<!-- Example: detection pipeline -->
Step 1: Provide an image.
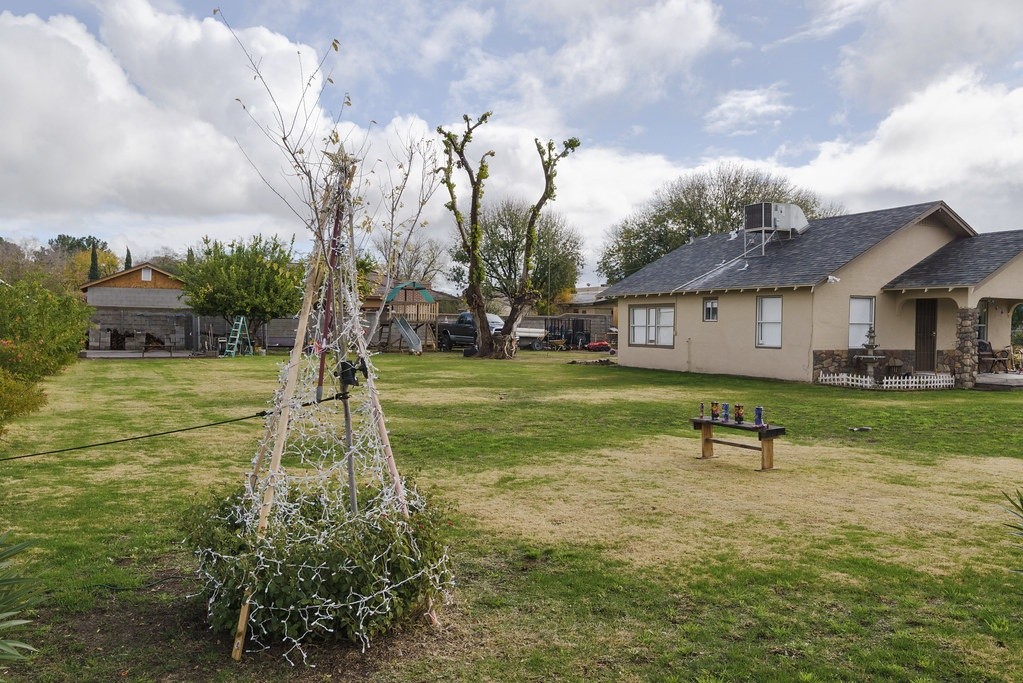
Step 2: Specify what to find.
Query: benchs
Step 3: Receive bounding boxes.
[139,345,176,358]
[689,416,785,470]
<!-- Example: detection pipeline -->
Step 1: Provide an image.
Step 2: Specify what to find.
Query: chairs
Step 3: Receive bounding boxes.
[978,339,1008,374]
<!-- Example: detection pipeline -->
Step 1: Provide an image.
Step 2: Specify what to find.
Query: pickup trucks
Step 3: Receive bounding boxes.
[430,312,519,357]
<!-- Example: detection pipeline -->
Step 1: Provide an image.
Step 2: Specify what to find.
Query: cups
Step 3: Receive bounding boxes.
[721,403,730,422]
[711,402,720,421]
[735,404,744,424]
[754,406,763,425]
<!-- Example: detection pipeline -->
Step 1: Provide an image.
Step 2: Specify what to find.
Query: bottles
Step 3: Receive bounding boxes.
[699,402,704,420]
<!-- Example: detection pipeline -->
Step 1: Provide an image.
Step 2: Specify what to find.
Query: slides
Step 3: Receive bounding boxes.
[392,316,422,350]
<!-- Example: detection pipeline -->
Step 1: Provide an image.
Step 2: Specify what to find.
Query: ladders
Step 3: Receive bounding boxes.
[223,315,252,358]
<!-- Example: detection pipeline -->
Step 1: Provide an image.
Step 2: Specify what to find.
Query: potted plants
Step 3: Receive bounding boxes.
[944,352,955,378]
[885,357,903,376]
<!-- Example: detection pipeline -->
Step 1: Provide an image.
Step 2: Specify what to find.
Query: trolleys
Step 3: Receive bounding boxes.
[549,338,570,351]
